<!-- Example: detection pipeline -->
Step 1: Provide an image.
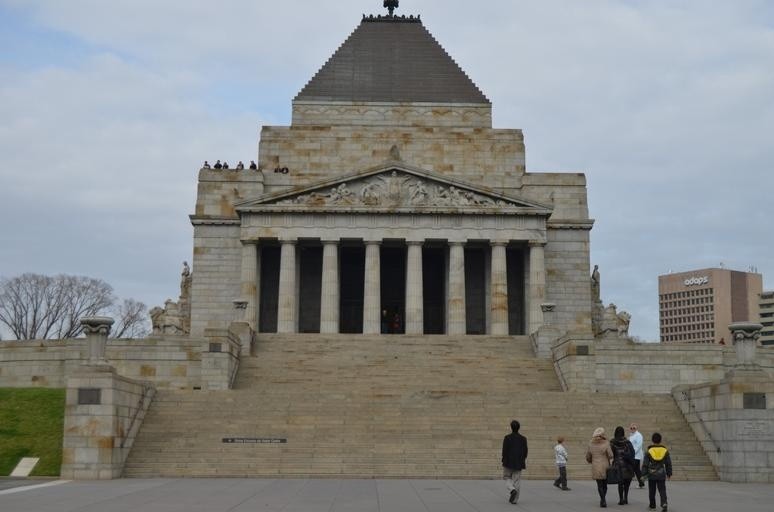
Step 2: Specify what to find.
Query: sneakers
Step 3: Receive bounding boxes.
[509,489,518,504]
[552,482,572,491]
[617,498,629,506]
[661,504,668,512]
[648,504,656,511]
[599,499,608,508]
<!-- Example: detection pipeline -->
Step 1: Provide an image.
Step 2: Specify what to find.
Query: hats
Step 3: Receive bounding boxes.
[593,427,605,438]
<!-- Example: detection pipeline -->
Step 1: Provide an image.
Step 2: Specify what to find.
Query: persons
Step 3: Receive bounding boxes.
[380,308,389,334]
[641,432,673,509]
[609,425,636,505]
[501,419,528,504]
[293,171,517,209]
[390,311,401,330]
[626,422,645,489]
[585,426,615,508]
[202,160,289,174]
[180,261,189,295]
[591,265,601,303]
[553,436,572,492]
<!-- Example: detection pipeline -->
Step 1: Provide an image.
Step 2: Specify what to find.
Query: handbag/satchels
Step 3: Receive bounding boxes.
[605,456,622,485]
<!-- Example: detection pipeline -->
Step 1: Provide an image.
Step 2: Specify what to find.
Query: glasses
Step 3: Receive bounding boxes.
[630,426,636,430]
